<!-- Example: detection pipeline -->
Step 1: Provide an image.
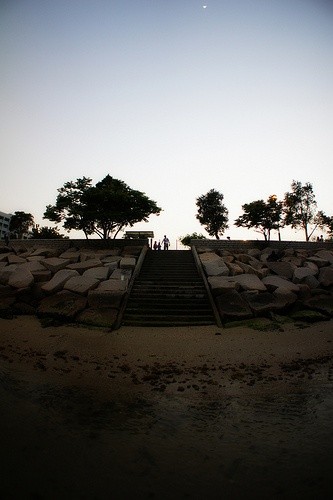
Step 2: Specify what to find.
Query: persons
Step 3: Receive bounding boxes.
[157,242,161,250]
[153,241,157,250]
[161,235,170,252]
